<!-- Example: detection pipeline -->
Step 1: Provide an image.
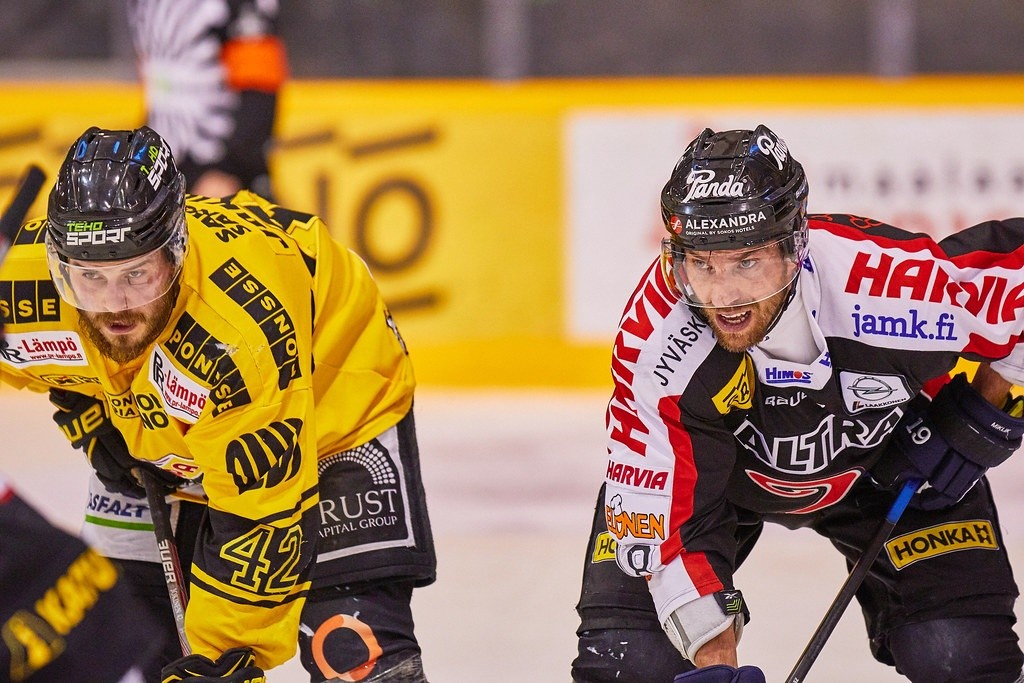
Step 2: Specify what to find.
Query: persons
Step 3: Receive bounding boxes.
[0,126,438,683]
[571,124,1024,682]
[126,0,289,205]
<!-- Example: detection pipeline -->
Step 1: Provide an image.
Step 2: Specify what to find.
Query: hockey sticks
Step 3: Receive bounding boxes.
[784,479,917,683]
[142,464,189,657]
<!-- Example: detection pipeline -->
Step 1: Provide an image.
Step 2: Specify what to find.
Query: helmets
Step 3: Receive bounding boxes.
[47,125,187,260]
[661,124,809,250]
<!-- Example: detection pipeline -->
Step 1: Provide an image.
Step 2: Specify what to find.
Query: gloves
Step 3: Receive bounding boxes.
[160,647,266,683]
[674,664,766,683]
[870,372,1024,511]
[48,387,190,499]
